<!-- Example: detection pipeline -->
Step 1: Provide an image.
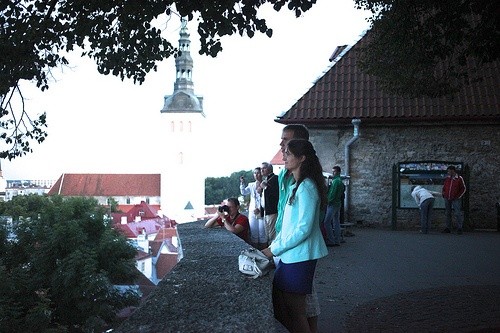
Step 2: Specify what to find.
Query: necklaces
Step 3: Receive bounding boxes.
[230,212,240,224]
[267,174,275,182]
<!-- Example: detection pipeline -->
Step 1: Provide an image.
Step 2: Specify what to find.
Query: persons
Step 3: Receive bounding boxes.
[240,162,279,269]
[411,185,435,234]
[261,125,327,333]
[325,165,345,247]
[442,165,467,235]
[205,198,249,242]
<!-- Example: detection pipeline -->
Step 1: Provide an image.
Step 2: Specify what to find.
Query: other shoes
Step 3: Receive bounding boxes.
[445,227,451,233]
[419,228,428,234]
[457,228,462,234]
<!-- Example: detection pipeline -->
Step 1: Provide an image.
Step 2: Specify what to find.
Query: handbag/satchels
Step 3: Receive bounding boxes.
[237,248,271,279]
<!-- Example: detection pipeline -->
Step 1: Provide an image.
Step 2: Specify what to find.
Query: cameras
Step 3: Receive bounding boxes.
[218,205,229,213]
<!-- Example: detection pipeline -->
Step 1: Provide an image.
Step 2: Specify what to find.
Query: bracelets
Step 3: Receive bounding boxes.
[222,219,227,222]
[264,184,268,190]
